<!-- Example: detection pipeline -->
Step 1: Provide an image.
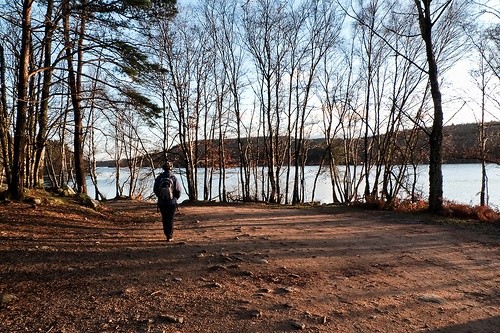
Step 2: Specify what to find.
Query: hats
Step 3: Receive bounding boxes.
[163,162,173,170]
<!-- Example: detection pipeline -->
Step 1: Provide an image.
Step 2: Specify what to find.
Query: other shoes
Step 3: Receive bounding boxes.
[167,236,174,242]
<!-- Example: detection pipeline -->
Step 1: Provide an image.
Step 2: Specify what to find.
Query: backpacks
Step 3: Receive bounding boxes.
[159,173,174,200]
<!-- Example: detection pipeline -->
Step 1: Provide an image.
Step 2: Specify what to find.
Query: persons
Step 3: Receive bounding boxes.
[153,160,181,241]
